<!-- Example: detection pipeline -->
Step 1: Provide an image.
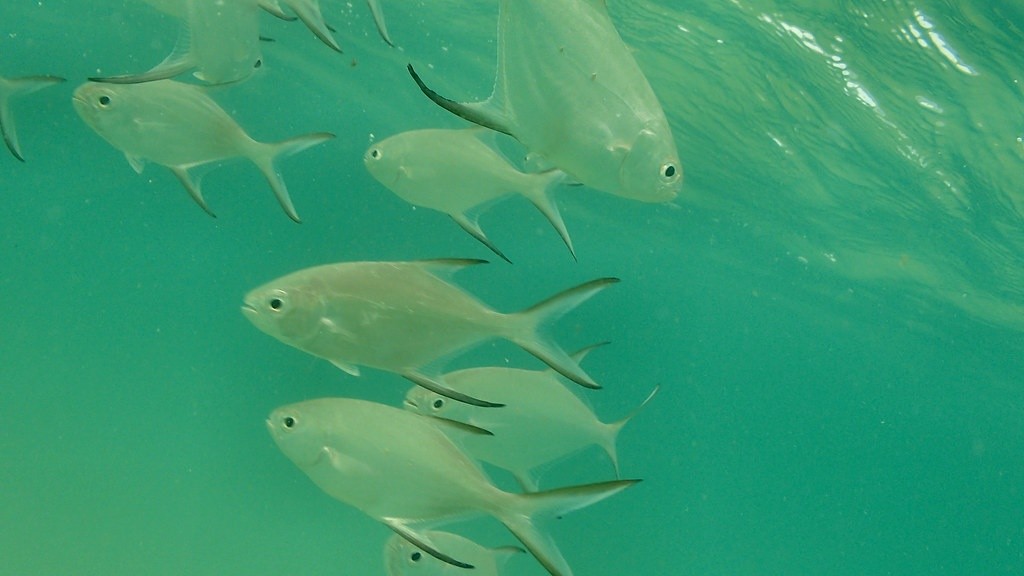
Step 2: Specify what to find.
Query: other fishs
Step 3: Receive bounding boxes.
[242,258,659,576]
[364,0,682,266]
[0,0,396,222]
